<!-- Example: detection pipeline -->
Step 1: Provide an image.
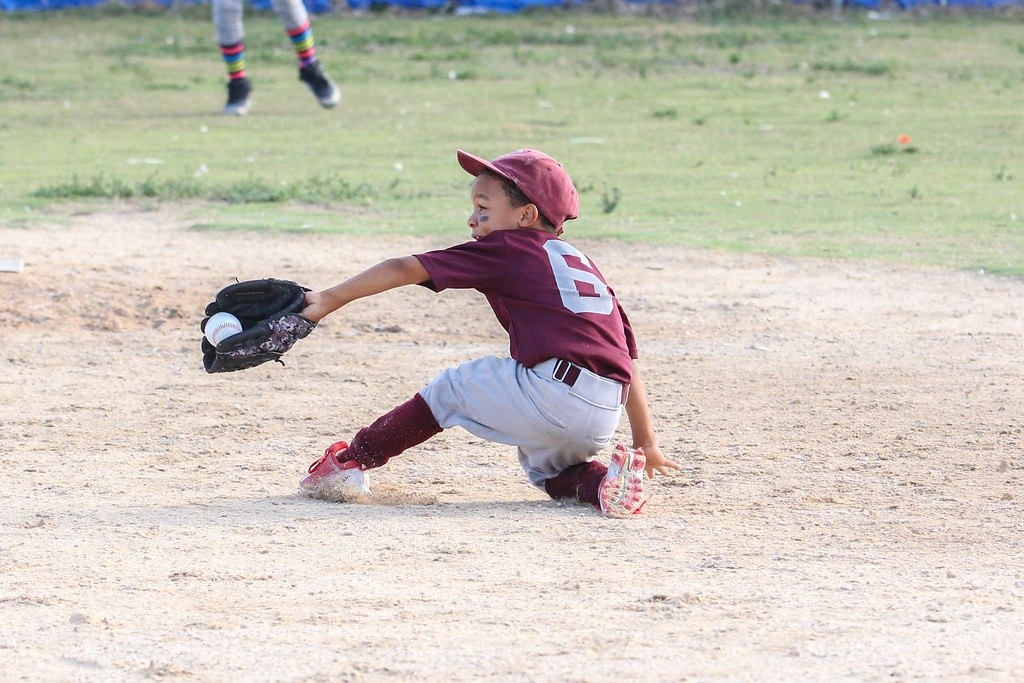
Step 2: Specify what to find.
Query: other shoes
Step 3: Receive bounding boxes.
[224,75,255,117]
[299,59,341,108]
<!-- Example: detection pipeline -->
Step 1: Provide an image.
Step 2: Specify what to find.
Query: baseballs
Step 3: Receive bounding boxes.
[205,311,246,349]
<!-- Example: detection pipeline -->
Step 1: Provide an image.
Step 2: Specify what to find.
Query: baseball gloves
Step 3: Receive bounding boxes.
[200,278,320,372]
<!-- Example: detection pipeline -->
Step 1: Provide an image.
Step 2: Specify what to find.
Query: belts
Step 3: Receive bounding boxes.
[552,359,629,404]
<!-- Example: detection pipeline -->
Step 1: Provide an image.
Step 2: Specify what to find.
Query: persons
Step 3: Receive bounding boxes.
[200,149,685,517]
[212,0,341,116]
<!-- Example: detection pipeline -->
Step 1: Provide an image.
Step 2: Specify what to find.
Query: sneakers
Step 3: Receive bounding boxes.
[299,442,368,501]
[598,444,646,518]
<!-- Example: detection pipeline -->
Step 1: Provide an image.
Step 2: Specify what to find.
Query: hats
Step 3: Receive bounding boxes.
[457,149,579,236]
